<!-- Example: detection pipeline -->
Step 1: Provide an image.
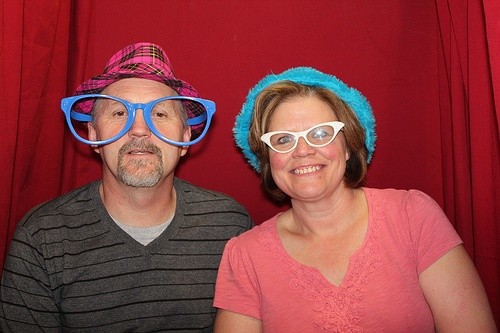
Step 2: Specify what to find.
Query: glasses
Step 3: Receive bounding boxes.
[61,94,216,146]
[260,121,344,153]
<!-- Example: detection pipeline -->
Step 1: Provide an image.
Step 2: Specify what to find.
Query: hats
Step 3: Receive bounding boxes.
[231,66,375,174]
[71,42,206,130]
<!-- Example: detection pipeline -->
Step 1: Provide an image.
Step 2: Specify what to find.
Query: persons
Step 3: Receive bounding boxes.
[211,67,499,333]
[0,43,255,333]
